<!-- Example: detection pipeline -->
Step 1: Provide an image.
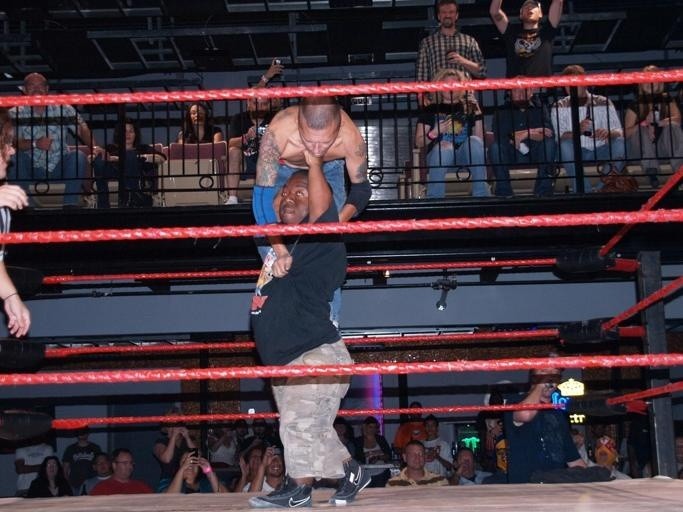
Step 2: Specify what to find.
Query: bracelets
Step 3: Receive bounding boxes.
[4,291,17,302]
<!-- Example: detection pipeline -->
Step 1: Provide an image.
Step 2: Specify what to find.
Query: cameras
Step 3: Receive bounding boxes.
[258,126,267,136]
[273,447,283,455]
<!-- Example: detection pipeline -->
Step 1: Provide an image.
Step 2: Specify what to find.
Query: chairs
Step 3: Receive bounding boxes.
[4,132,256,209]
[394,129,682,202]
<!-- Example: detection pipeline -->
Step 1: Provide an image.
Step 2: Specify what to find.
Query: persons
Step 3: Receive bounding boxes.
[15,422,285,499]
[253,97,371,509]
[676,436,683,477]
[1,103,31,337]
[6,58,289,217]
[416,0,563,204]
[333,345,632,488]
[551,63,683,188]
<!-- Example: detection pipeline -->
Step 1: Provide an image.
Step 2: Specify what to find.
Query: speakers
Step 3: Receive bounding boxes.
[157,159,221,207]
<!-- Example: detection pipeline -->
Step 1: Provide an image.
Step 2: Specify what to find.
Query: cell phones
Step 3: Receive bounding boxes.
[188,447,198,465]
[274,59,283,72]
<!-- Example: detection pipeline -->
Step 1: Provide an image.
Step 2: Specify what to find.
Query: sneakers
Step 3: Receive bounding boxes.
[328,460,371,507]
[248,473,312,509]
